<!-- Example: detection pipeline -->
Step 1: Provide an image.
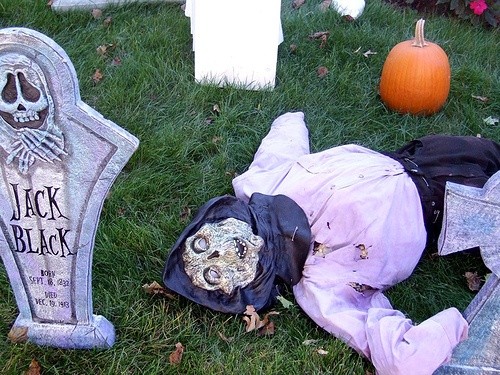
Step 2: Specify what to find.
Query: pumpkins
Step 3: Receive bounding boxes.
[379,18,451,117]
